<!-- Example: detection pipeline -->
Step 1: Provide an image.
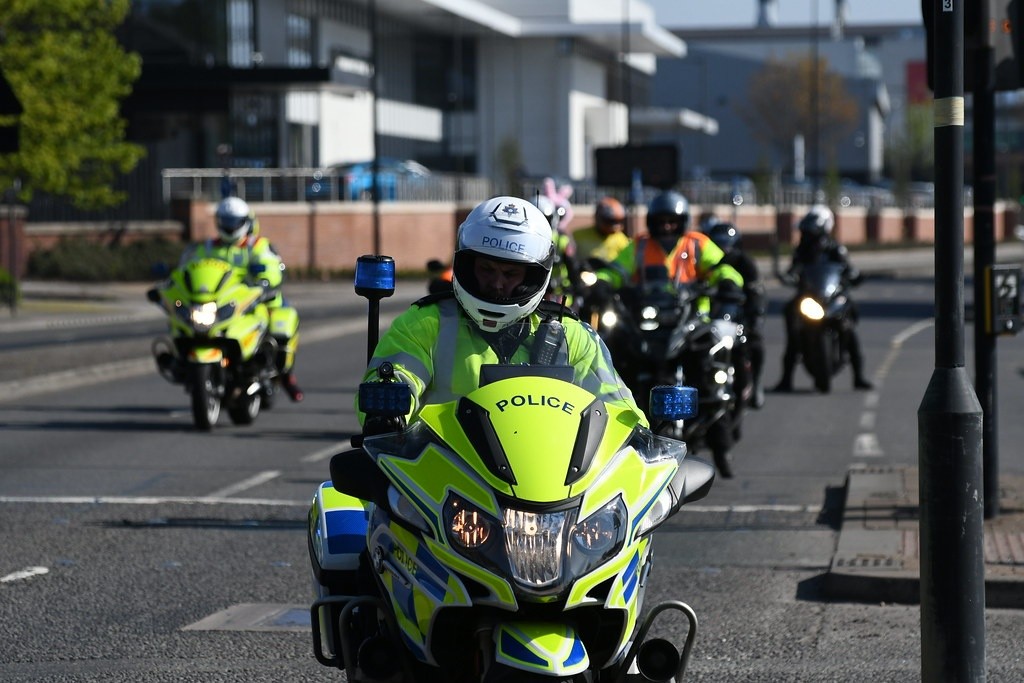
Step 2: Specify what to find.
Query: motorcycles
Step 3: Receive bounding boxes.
[537,222,765,483]
[769,248,869,392]
[304,254,743,683]
[140,252,304,435]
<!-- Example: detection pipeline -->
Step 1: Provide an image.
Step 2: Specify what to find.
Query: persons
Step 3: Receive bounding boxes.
[692,205,767,412]
[570,194,634,330]
[770,202,878,395]
[158,195,306,407]
[581,187,740,480]
[353,196,669,500]
[530,173,586,319]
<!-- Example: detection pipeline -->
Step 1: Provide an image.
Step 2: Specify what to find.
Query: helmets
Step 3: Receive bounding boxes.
[452,196,555,332]
[709,223,742,248]
[647,191,691,241]
[214,196,250,243]
[595,198,625,220]
[698,212,719,232]
[799,204,835,233]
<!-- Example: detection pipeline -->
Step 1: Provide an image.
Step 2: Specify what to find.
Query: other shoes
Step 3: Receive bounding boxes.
[713,450,737,477]
[749,385,764,406]
[772,382,794,392]
[854,377,873,389]
[281,377,305,402]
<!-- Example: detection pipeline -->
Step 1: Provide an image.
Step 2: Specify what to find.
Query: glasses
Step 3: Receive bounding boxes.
[596,214,625,225]
